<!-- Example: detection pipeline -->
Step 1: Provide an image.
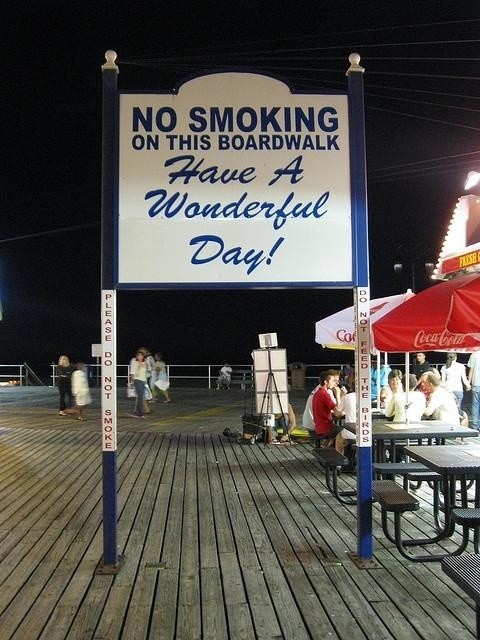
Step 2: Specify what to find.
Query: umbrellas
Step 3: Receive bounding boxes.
[315,288,417,493]
[371,272,480,353]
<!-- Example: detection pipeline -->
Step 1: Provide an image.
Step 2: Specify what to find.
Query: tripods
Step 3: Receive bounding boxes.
[254,350,291,449]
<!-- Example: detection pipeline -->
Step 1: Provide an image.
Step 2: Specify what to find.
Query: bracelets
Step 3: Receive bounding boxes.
[286,434,288,436]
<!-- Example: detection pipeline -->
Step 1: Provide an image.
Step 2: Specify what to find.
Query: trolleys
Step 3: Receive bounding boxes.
[237,373,265,446]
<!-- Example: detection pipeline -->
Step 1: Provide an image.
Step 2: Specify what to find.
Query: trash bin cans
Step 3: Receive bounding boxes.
[289,363,306,390]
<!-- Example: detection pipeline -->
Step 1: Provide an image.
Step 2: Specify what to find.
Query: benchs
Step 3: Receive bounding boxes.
[441,554,480,640]
[308,401,480,563]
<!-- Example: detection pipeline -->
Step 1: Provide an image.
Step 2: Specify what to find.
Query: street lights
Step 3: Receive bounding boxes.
[391,261,433,293]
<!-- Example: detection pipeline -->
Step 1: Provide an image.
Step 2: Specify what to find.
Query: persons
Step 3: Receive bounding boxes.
[126,347,171,419]
[57,355,74,416]
[267,404,296,443]
[302,352,480,471]
[71,359,92,422]
[215,362,232,390]
